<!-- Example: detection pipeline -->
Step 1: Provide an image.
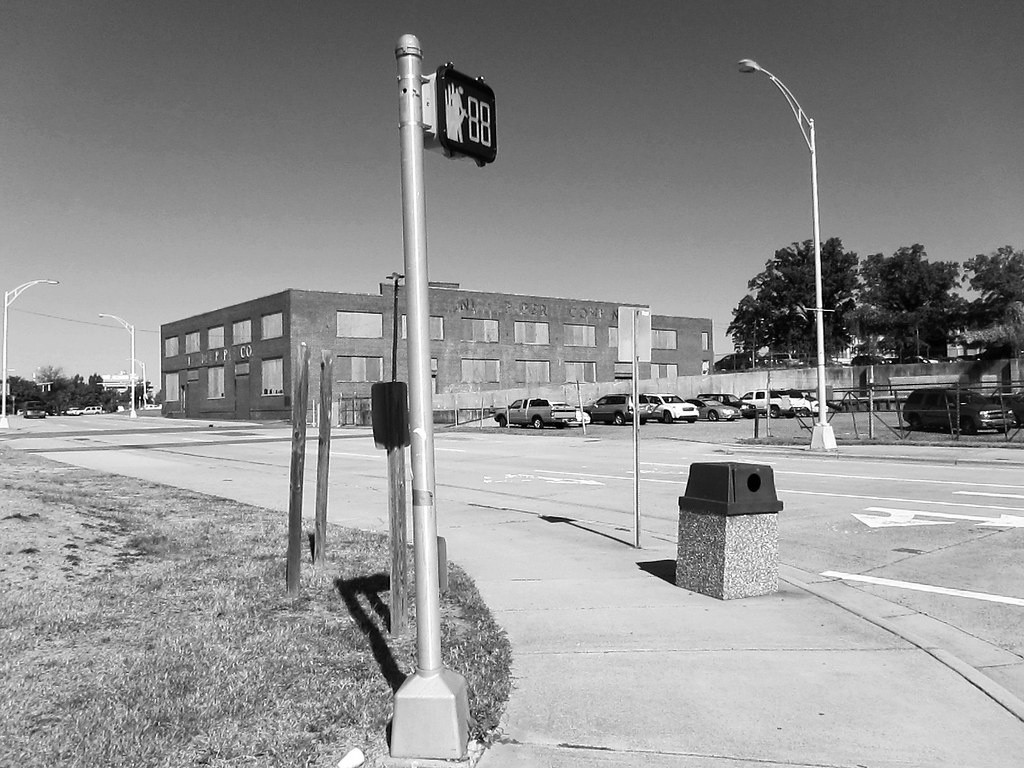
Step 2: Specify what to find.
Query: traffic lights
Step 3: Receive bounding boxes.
[431,64,498,169]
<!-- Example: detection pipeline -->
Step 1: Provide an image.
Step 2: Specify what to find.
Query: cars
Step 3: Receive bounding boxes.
[65,408,79,415]
[140,404,157,410]
[552,401,591,427]
[1002,391,1024,429]
[684,398,742,421]
[17,409,23,416]
[75,406,102,416]
[804,397,828,417]
[902,388,1017,436]
[696,394,757,419]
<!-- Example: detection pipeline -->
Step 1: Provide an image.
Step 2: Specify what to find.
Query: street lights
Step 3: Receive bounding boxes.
[127,358,147,408]
[98,312,137,419]
[737,58,837,449]
[0,280,58,429]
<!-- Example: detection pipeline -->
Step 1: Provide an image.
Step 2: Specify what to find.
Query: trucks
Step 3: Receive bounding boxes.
[22,401,46,419]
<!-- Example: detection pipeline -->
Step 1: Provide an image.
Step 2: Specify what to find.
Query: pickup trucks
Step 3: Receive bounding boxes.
[739,389,806,419]
[494,398,576,430]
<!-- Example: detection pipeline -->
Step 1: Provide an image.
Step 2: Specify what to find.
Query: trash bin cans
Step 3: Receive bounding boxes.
[674,463,786,601]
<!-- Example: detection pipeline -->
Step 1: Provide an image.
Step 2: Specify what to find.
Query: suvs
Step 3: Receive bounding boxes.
[583,393,654,426]
[642,393,700,423]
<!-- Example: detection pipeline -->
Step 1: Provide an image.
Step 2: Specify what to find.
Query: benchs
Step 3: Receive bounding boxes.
[842,396,906,411]
